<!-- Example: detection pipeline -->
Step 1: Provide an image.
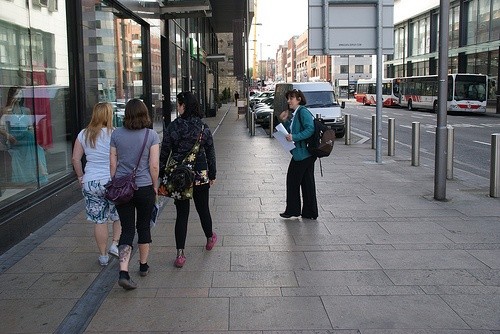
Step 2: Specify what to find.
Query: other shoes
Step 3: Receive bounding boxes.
[206,231,218,251]
[140,262,150,275]
[119,270,136,290]
[175,255,186,268]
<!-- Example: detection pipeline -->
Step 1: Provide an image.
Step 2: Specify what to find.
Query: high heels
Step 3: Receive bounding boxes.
[300,214,318,220]
[279,212,293,218]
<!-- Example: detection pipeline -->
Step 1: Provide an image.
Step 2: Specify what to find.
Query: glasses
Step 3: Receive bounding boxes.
[293,88,298,92]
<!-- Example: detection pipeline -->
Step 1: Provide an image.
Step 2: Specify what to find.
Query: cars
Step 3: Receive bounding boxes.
[250,87,274,129]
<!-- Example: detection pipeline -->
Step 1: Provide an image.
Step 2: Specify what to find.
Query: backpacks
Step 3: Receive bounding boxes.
[298,107,335,176]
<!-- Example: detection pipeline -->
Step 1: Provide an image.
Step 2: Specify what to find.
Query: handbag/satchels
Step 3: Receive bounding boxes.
[157,157,194,199]
[151,197,169,227]
[102,170,139,204]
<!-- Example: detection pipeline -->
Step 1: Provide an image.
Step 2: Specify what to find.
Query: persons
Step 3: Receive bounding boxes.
[72,102,119,266]
[159,92,217,267]
[235,90,240,106]
[0,47,54,188]
[110,98,162,288]
[280,90,319,220]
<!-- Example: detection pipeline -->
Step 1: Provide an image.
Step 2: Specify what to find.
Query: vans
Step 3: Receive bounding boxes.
[273,81,346,138]
[5,85,72,147]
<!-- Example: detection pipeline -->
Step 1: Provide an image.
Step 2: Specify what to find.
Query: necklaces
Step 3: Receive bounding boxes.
[11,105,24,120]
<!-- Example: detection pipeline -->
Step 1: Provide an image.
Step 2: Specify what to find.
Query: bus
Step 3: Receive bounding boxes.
[354,73,488,114]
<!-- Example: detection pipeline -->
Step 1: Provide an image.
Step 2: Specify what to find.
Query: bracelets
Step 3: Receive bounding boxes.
[7,133,9,141]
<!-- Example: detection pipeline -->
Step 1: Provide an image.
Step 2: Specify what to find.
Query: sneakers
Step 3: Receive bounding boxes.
[109,244,119,259]
[99,253,109,265]
